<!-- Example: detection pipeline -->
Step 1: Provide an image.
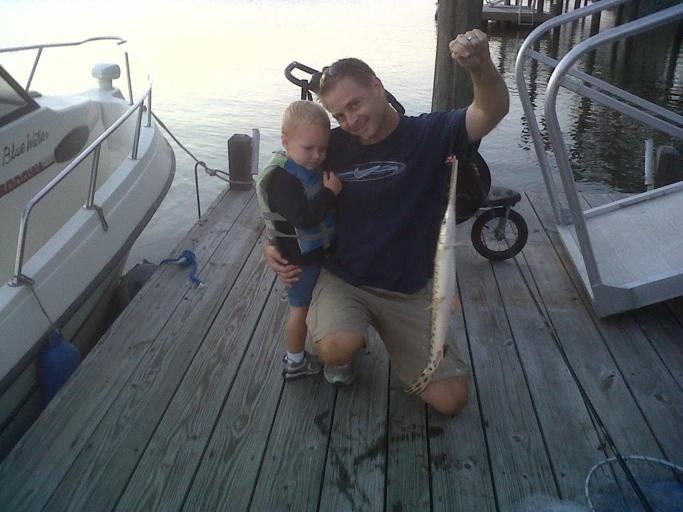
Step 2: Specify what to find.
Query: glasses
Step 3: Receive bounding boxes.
[318,61,345,90]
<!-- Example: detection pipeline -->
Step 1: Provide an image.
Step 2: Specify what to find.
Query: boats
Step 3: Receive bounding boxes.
[0,33,176,474]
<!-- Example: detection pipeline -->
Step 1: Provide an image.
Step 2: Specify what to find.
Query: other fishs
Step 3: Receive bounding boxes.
[403,159,458,396]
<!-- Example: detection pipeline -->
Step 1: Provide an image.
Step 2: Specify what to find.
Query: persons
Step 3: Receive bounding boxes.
[255,99,344,380]
[266,29,509,416]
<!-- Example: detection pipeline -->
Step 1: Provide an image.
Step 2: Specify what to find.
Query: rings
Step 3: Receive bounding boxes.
[467,36,477,41]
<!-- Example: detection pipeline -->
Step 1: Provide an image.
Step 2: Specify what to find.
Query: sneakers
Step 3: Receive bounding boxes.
[323,361,359,387]
[281,348,322,381]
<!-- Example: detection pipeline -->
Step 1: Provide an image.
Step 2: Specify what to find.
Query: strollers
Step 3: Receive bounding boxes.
[281,58,527,264]
